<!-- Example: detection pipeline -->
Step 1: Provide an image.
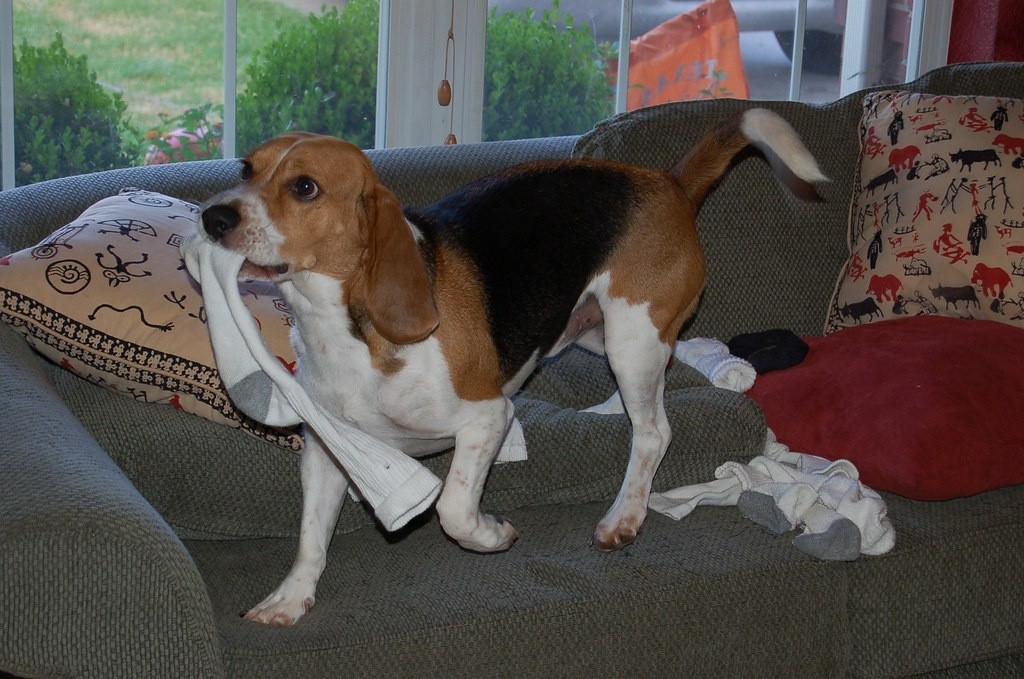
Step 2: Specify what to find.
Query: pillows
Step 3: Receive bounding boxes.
[823,92,1024,338]
[422,350,769,520]
[0,186,305,456]
[57,367,376,539]
[744,314,1024,503]
[573,61,1024,340]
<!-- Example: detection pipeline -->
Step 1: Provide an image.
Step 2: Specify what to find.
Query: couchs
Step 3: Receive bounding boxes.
[0,134,1024,678]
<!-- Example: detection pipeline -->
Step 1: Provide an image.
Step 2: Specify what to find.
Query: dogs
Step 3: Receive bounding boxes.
[199,107,833,628]
